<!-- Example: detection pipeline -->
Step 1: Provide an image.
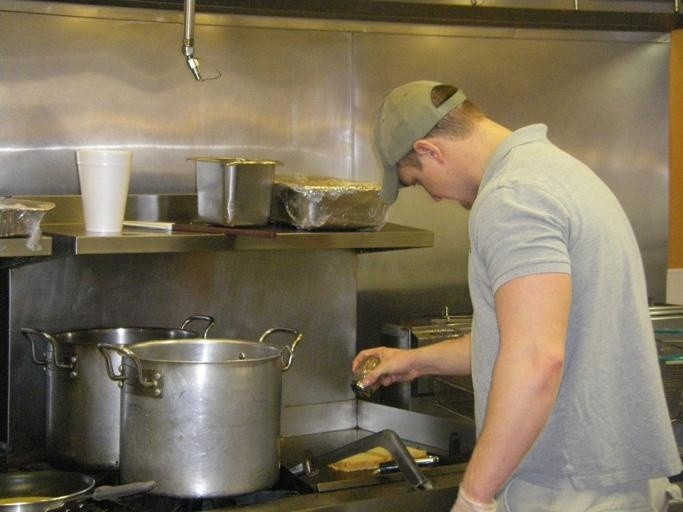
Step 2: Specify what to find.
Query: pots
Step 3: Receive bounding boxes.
[18,315,222,471]
[95,327,303,501]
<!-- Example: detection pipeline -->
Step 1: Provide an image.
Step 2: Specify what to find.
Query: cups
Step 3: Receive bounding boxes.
[75,149,133,235]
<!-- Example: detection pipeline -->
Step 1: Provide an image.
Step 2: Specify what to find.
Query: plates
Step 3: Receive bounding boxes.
[0,468,160,512]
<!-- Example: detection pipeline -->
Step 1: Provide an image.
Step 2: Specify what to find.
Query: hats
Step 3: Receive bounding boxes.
[370,79,467,204]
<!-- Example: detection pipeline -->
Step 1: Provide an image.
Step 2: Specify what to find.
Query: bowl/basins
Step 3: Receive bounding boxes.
[187,155,280,229]
[0,197,57,234]
[274,180,387,229]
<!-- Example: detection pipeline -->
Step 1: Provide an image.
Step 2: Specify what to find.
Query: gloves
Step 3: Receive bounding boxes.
[450,482,499,511]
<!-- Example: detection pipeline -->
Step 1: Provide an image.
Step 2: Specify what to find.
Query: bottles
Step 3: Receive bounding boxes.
[351,355,385,399]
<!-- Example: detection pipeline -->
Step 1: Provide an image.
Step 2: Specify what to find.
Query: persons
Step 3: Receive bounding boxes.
[352,80,683,512]
